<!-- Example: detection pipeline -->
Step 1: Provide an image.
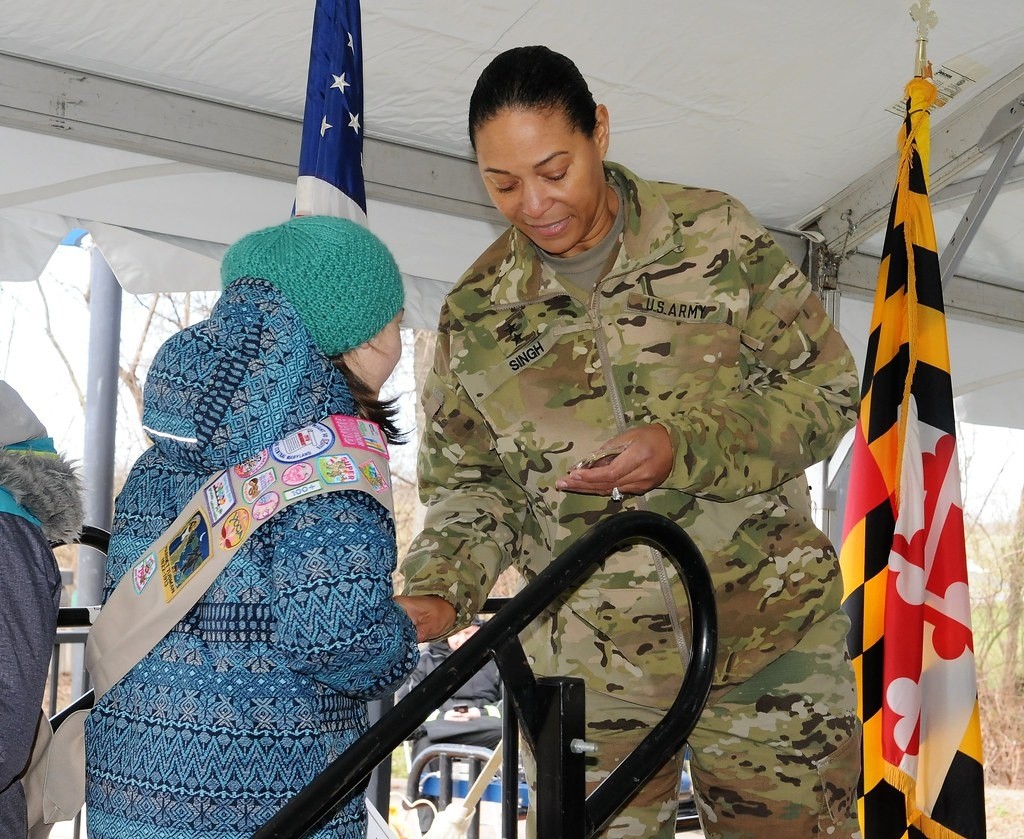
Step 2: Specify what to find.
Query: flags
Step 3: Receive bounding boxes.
[291,0,367,225]
[837,80,987,839]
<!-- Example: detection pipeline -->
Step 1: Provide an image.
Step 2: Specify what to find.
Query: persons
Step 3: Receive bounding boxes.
[389,45,860,839]
[0,380,83,839]
[84,216,420,839]
[395,618,504,833]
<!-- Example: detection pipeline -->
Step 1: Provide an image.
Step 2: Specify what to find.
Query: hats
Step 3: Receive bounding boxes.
[221,215,405,358]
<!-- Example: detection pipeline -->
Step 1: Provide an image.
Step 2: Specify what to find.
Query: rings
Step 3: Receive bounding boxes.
[610,487,623,501]
